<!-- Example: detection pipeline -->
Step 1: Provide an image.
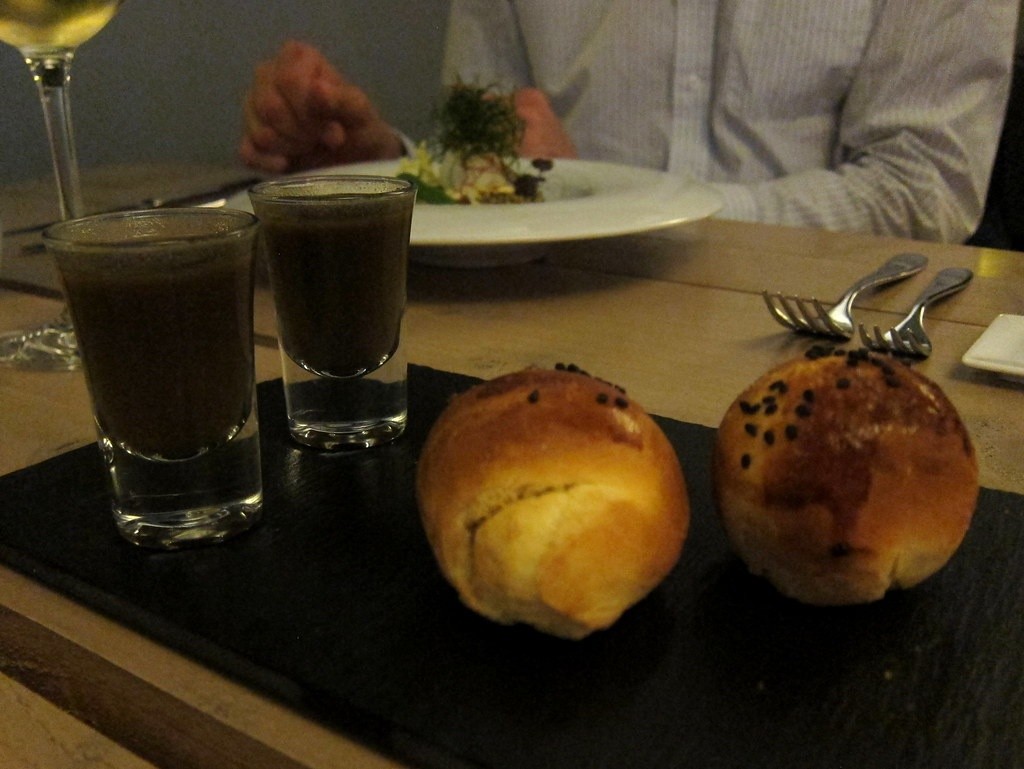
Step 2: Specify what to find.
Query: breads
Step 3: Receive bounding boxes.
[716,344,979,606]
[417,361,690,640]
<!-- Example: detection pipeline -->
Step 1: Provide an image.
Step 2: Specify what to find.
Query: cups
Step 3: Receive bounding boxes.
[249,172,418,449]
[41,206,265,550]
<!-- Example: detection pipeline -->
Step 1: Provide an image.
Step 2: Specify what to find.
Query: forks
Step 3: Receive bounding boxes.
[860,267,976,358]
[762,253,928,339]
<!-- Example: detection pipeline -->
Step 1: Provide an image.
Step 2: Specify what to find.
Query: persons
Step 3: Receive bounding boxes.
[239,0,1019,243]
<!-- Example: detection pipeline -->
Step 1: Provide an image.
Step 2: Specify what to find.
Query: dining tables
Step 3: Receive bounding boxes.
[0,157,1024,769]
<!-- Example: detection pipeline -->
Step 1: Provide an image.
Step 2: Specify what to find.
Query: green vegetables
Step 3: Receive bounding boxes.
[394,75,526,205]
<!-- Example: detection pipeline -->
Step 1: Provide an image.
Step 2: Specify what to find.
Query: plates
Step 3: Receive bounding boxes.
[230,157,721,270]
[963,314,1024,385]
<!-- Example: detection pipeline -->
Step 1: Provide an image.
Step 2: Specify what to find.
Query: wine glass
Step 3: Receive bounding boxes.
[0,0,124,372]
